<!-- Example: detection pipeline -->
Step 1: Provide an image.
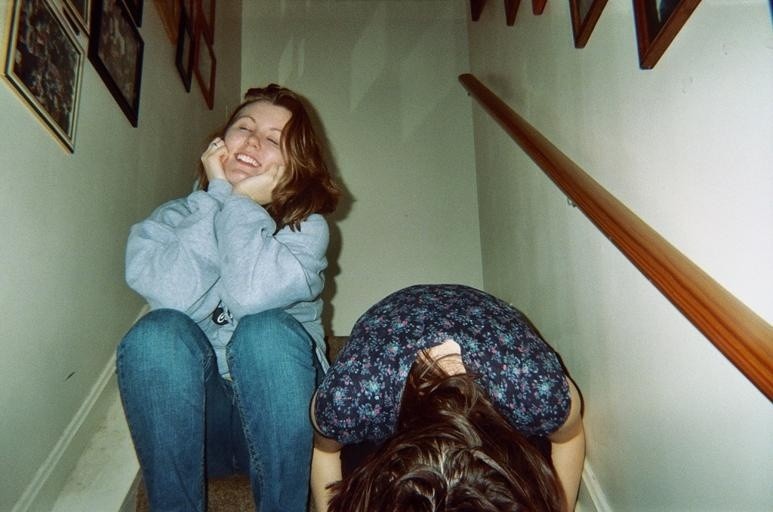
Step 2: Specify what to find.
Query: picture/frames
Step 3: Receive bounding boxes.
[0,0,85,156]
[470,1,703,72]
[60,0,218,128]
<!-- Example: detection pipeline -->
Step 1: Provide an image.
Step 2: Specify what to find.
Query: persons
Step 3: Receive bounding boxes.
[307,285,586,512]
[116,83,341,512]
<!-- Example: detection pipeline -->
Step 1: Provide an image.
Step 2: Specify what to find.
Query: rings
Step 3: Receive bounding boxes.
[213,143,218,148]
